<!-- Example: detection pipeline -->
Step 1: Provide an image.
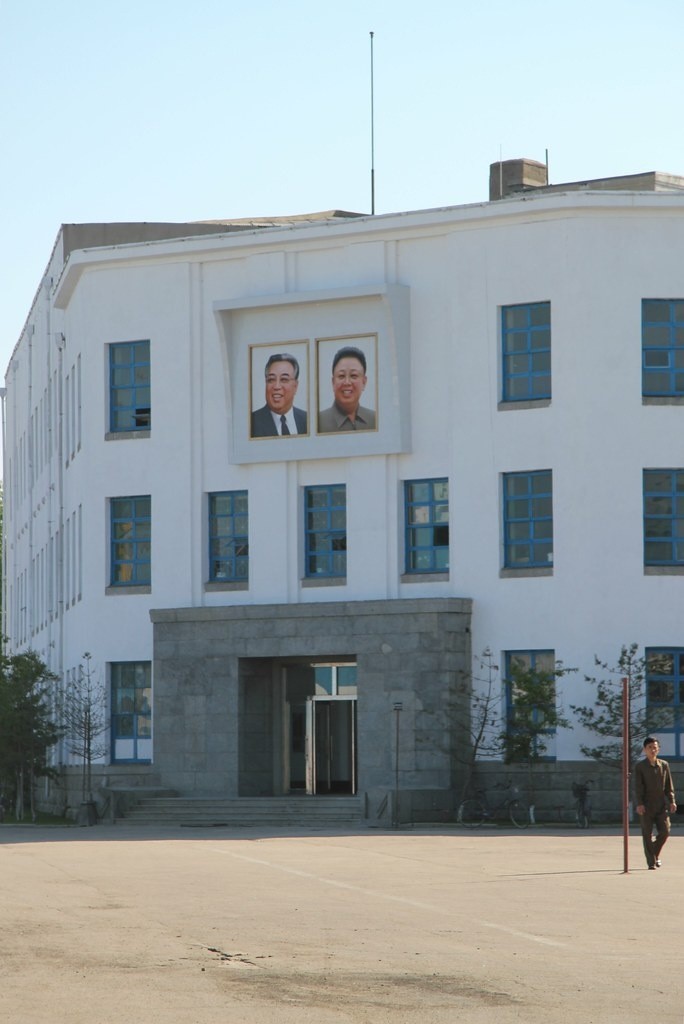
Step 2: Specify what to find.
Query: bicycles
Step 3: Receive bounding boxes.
[569,780,594,827]
[456,788,530,830]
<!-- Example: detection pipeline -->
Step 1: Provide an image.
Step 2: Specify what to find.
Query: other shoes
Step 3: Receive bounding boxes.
[655,859,662,867]
[649,865,654,869]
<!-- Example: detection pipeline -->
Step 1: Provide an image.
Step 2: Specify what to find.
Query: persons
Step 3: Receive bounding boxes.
[319,347,375,432]
[635,737,677,869]
[252,354,307,438]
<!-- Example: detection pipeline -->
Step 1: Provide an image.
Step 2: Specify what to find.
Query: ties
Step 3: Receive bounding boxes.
[280,415,291,435]
